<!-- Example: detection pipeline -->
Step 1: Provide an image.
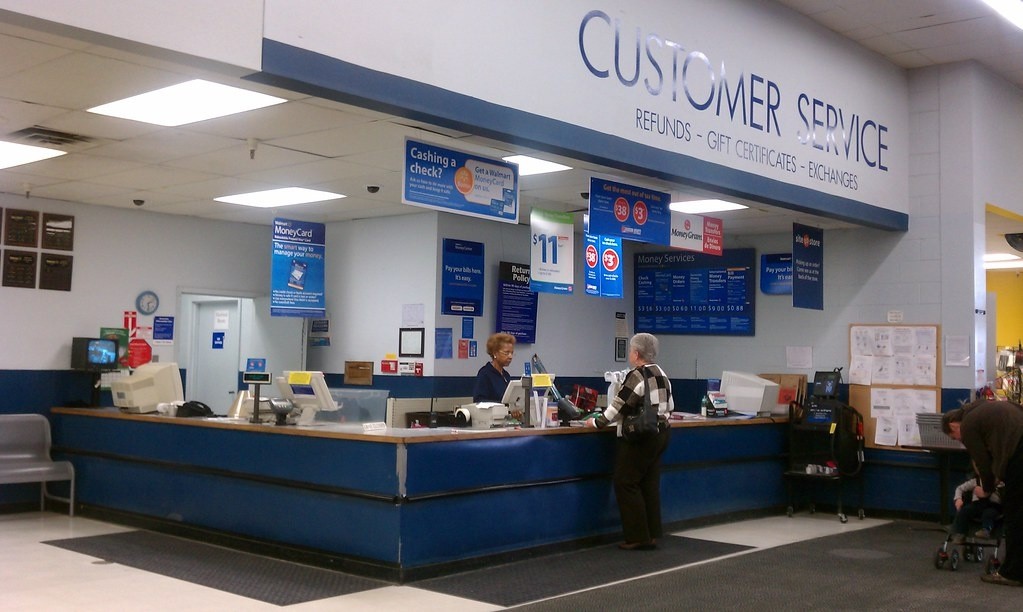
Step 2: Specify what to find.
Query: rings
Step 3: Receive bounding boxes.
[586,421,589,424]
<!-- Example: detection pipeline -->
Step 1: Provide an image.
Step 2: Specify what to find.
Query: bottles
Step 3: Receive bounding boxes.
[701,396,708,417]
[542,402,558,427]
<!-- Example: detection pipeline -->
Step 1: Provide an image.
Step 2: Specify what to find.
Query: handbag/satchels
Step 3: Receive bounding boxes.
[605,368,629,406]
[621,365,657,443]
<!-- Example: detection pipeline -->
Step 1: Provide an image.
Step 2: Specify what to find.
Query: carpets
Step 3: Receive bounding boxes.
[500,520,1023,612]
[40,530,394,607]
[405,533,758,608]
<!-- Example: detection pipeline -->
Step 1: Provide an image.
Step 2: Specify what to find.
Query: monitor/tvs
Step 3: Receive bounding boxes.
[110,362,183,413]
[719,370,779,417]
[275,371,336,411]
[71,337,119,370]
[502,374,556,414]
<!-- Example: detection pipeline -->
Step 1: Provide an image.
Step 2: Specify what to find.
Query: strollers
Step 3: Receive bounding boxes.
[934,473,1005,575]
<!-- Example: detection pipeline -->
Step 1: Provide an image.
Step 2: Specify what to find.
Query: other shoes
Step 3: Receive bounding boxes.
[976,528,990,538]
[952,533,967,544]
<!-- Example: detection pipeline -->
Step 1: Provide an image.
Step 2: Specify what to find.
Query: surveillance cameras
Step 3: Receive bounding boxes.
[366,184,379,193]
[133,199,144,205]
[580,192,589,200]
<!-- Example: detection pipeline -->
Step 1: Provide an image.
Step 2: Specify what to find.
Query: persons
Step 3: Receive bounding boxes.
[942,400,1023,587]
[473,333,522,420]
[584,333,674,549]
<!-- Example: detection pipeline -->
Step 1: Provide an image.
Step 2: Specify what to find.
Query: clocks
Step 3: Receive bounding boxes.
[136,290,159,314]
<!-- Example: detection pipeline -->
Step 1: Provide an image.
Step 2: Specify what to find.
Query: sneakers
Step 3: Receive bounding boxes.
[981,572,1020,586]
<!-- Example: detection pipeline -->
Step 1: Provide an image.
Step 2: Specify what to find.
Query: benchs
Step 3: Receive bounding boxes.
[0,413,75,517]
